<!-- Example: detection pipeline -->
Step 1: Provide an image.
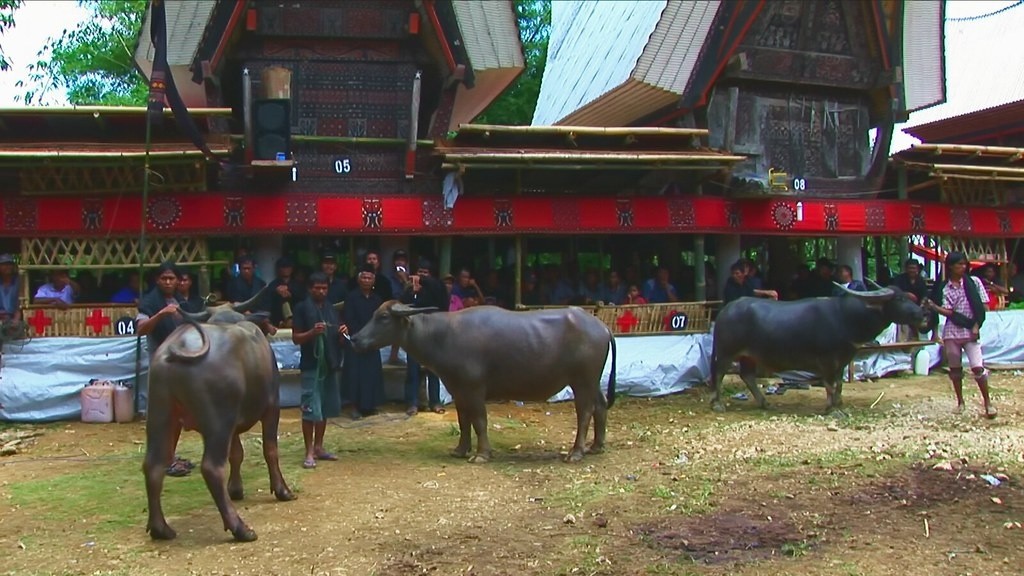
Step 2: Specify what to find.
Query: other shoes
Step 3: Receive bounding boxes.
[953,405,966,413]
[352,409,362,418]
[765,386,777,394]
[776,387,786,394]
[166,462,191,477]
[734,393,749,400]
[787,382,810,389]
[407,405,418,416]
[431,404,446,414]
[372,408,380,414]
[175,457,196,469]
[984,406,998,416]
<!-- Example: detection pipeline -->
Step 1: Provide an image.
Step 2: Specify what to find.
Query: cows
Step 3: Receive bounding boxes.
[711,275,939,413]
[140,287,297,542]
[353,301,617,464]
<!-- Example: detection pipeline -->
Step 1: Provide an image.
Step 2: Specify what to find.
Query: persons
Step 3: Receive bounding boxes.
[0,249,1024,416]
[929,252,997,417]
[292,273,349,468]
[135,263,194,477]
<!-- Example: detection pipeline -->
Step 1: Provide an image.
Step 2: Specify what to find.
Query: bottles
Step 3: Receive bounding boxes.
[915,349,930,376]
[276,153,285,163]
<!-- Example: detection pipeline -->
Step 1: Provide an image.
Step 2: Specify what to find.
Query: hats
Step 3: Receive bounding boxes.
[1,253,14,263]
[442,274,455,282]
[359,264,375,272]
[322,252,337,260]
[394,249,408,256]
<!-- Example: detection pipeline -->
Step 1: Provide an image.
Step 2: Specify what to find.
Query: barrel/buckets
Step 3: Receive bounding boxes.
[81,380,115,424]
[113,380,136,422]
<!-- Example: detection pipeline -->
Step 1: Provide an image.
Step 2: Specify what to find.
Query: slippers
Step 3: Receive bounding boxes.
[313,453,339,460]
[303,460,317,469]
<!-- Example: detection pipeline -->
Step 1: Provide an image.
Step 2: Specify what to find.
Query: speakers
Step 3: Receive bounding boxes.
[253,99,289,161]
